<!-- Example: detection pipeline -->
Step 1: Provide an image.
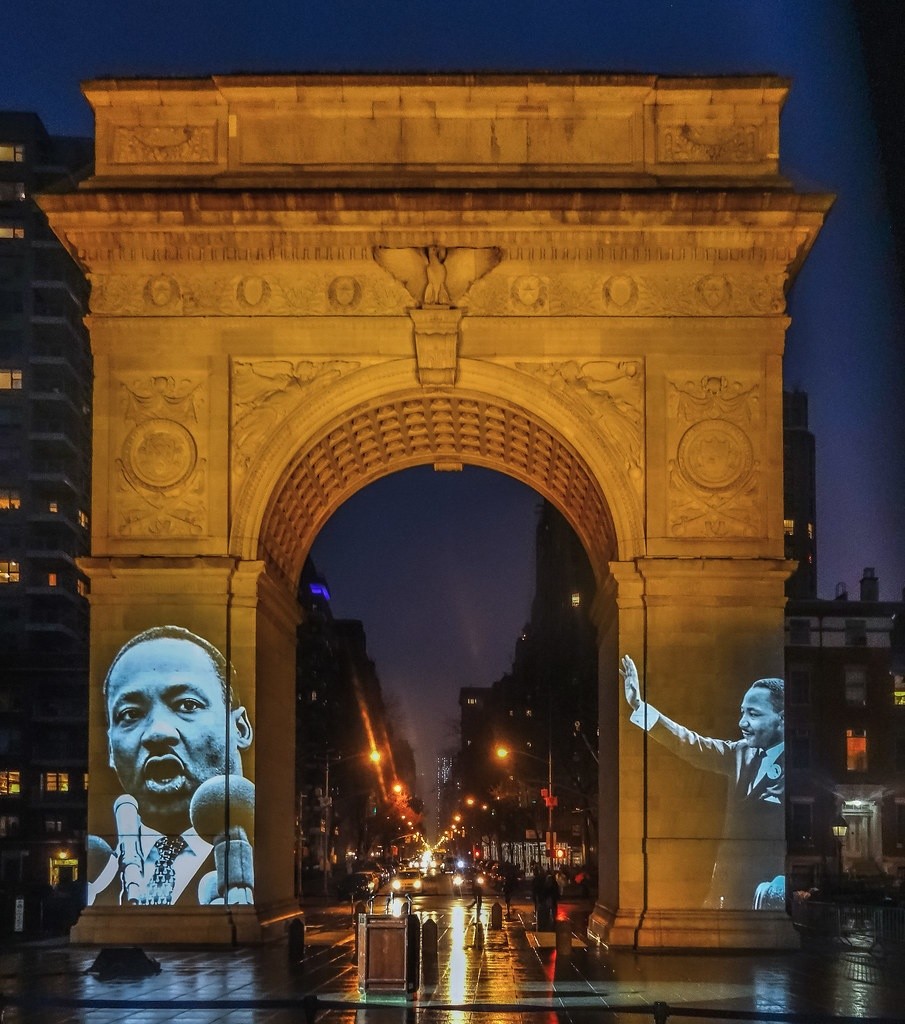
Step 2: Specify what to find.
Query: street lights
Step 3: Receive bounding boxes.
[321,750,381,892]
[830,812,848,927]
[497,748,555,873]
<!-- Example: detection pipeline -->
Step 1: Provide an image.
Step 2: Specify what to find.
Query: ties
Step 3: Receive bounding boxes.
[139,836,188,905]
[736,747,767,800]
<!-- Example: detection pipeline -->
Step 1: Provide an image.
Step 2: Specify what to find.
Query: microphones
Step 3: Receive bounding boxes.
[114,794,143,902]
[191,773,255,904]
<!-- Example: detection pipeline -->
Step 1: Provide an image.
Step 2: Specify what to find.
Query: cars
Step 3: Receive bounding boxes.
[393,869,424,894]
[347,872,379,897]
[451,867,488,896]
[439,856,520,892]
[362,853,437,887]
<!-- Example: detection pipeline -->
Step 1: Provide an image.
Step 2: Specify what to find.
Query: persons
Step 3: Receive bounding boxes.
[503,875,513,918]
[529,859,590,932]
[87,626,254,905]
[618,654,786,911]
[466,874,483,922]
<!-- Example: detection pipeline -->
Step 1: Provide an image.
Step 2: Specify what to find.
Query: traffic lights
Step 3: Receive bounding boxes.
[554,848,565,857]
